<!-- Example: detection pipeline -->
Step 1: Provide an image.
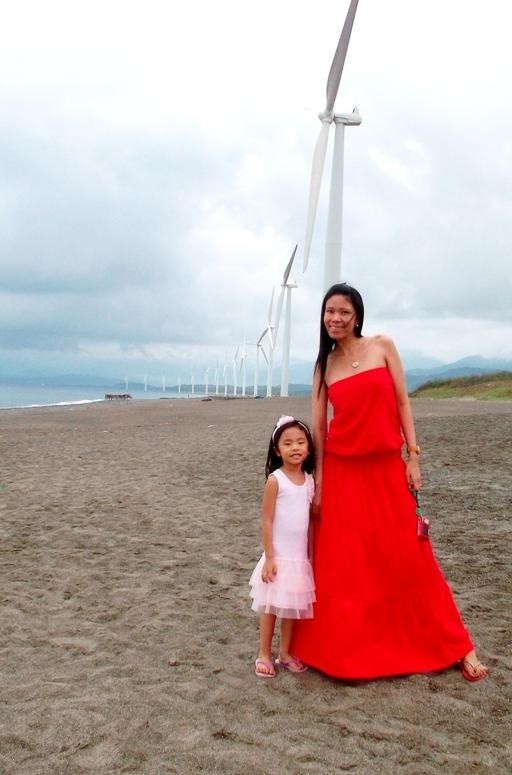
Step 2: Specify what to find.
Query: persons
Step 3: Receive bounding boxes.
[248,413,320,676]
[289,282,490,682]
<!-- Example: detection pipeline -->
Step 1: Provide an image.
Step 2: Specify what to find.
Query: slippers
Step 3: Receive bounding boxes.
[461,657,489,681]
[255,657,308,677]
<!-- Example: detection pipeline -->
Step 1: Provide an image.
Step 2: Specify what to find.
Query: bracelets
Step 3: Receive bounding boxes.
[408,446,420,455]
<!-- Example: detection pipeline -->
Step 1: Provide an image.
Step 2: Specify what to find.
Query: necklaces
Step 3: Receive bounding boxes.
[343,339,370,368]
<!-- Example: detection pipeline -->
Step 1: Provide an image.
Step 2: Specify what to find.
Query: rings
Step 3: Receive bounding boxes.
[413,478,419,484]
